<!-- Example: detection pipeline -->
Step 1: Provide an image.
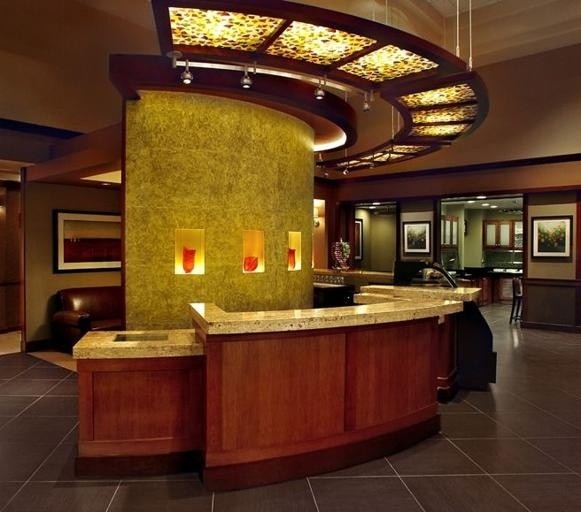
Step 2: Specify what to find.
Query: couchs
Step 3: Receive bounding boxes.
[54,284,127,351]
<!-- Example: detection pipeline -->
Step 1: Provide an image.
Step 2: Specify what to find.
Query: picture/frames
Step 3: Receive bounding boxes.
[531,215,574,261]
[403,221,434,256]
[51,207,122,273]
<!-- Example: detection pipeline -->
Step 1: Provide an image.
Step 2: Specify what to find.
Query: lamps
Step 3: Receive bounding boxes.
[180,61,374,114]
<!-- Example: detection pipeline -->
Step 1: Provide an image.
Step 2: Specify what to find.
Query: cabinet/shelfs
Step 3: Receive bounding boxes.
[484,217,513,248]
